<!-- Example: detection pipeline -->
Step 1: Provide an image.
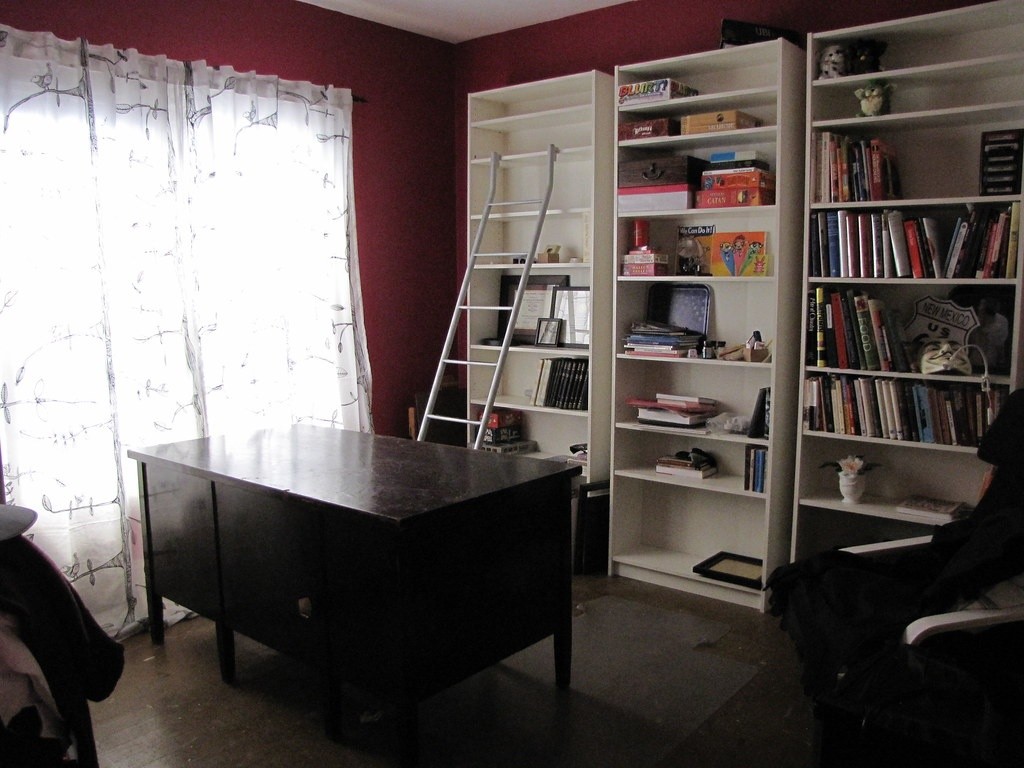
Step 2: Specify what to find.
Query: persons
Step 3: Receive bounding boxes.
[621,320,770,493]
[529,358,588,410]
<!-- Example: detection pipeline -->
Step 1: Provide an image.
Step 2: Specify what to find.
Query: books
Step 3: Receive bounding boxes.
[804,124,1020,446]
[896,489,966,521]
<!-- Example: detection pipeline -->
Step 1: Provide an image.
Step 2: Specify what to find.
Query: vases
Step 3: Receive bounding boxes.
[839,477,866,504]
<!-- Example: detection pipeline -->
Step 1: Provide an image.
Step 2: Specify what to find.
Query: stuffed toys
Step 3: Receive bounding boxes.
[815,42,843,79]
[853,79,897,117]
[847,38,887,75]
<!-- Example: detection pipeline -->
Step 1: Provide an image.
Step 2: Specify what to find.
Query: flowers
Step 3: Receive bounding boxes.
[816,447,889,477]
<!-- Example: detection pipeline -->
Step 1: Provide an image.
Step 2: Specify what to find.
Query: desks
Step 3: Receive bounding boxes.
[125,422,583,745]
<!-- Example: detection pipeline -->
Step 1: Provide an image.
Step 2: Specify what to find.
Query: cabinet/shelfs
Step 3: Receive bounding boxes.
[461,68,613,570]
[790,0,1024,601]
[605,34,804,617]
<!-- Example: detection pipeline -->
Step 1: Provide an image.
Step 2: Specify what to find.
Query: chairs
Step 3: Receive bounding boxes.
[798,536,1024,768]
